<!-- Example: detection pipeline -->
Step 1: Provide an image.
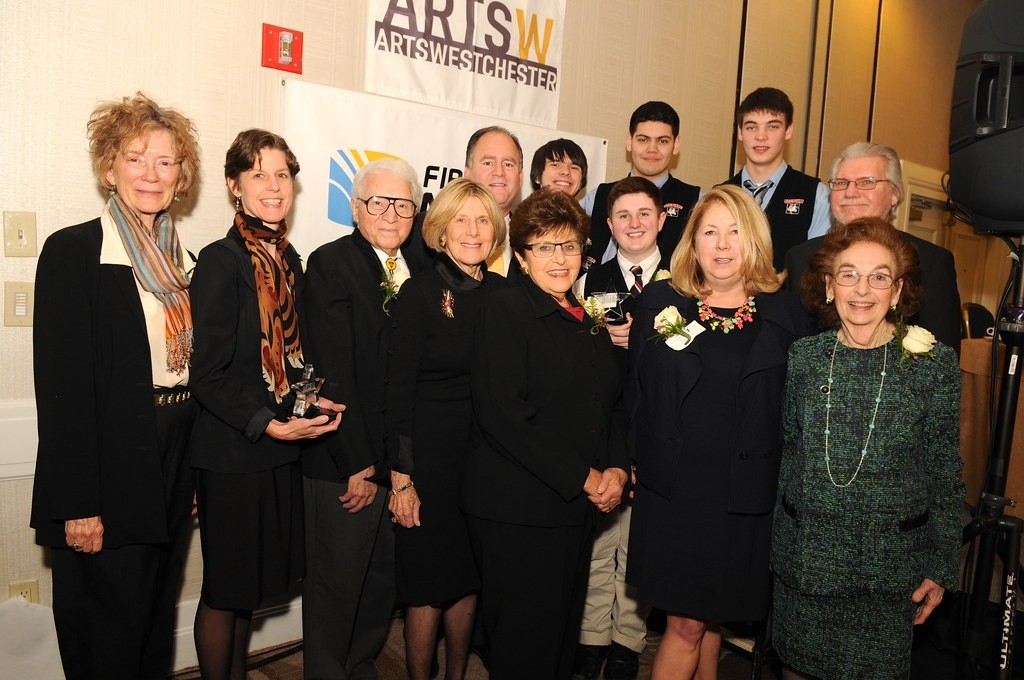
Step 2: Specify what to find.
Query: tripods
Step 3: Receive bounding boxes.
[911,233,1024,680]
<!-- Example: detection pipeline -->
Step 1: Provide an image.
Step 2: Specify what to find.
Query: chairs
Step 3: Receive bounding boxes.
[960,302,995,340]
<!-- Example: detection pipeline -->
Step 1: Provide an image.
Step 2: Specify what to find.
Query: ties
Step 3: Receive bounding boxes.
[629,265,643,298]
[743,180,774,207]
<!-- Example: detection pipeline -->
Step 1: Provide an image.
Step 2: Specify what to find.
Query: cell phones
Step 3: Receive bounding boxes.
[313,406,337,425]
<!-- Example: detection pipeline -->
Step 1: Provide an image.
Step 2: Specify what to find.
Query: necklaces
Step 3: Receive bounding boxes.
[697,285,756,334]
[824,334,887,489]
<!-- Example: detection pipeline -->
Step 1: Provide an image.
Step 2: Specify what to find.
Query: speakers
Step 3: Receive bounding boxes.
[948,0,1024,232]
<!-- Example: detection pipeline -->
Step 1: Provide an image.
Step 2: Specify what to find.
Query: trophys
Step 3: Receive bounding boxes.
[291,363,338,424]
[591,279,631,325]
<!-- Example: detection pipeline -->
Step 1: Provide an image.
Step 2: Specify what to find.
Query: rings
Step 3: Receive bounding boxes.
[391,517,398,523]
[609,505,612,509]
[72,544,79,549]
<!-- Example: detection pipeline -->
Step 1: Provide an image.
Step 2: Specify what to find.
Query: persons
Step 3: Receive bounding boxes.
[188,128,347,680]
[530,139,587,197]
[780,141,961,366]
[576,101,705,271]
[711,87,832,267]
[30,93,199,680]
[298,160,966,680]
[464,127,524,276]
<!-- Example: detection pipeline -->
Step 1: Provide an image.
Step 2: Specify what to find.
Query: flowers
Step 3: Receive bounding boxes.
[578,295,609,330]
[647,305,692,349]
[889,319,939,372]
[653,269,671,283]
[377,268,399,317]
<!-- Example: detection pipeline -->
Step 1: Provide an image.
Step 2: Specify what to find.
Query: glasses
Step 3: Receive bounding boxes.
[830,270,900,289]
[525,240,585,257]
[357,195,417,218]
[827,176,890,191]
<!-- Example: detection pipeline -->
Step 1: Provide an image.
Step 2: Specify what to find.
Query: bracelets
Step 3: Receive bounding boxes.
[388,481,414,496]
